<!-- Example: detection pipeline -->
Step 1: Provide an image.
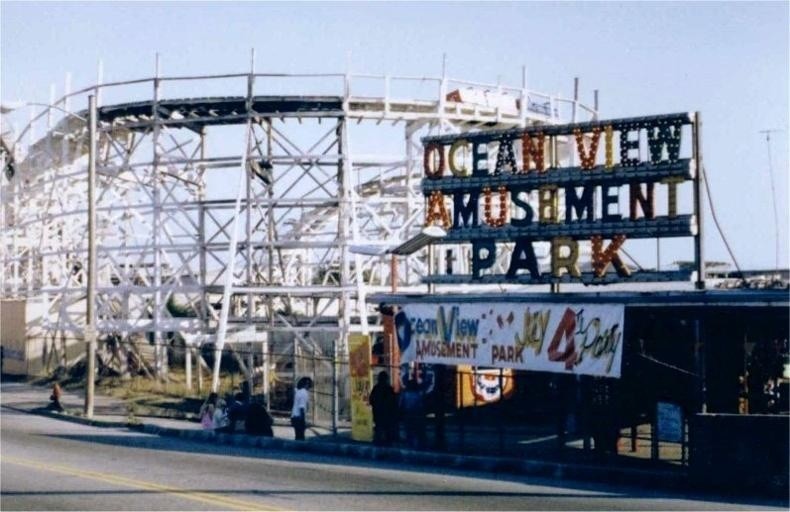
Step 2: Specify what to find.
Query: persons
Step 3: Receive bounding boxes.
[290,376,313,440]
[47,382,68,416]
[368,371,398,448]
[198,379,275,438]
[396,377,428,451]
